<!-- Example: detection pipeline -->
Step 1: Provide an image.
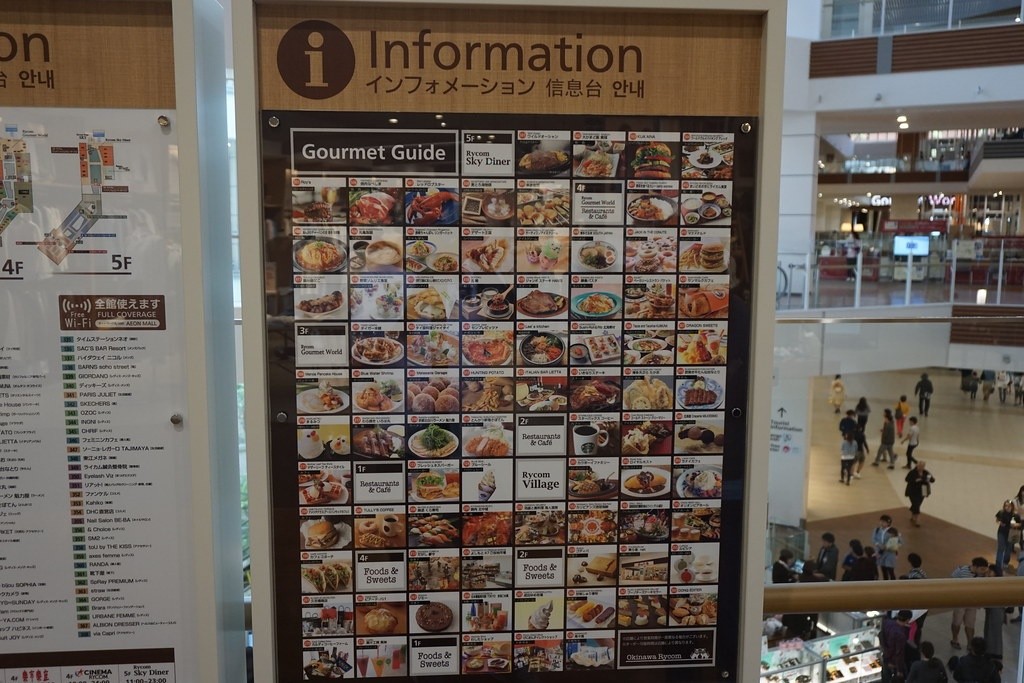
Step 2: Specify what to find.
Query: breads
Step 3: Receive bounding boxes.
[364,608,397,633]
[359,533,385,547]
[671,599,716,625]
[679,293,710,316]
[406,378,459,412]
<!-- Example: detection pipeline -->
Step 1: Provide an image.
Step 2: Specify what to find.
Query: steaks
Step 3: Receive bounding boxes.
[684,388,716,406]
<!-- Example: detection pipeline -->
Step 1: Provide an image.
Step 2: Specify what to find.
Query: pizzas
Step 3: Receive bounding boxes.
[462,340,512,365]
[465,244,504,272]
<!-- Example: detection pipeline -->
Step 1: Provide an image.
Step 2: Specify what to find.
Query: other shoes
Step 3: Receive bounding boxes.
[951,640,962,649]
[1010,616,1021,624]
[854,471,861,479]
[887,466,895,469]
[872,461,879,466]
[903,466,912,469]
[1004,607,1014,613]
[840,479,845,483]
[911,517,920,527]
[845,482,849,485]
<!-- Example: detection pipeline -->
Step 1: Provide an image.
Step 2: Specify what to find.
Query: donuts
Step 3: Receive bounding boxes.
[358,520,378,534]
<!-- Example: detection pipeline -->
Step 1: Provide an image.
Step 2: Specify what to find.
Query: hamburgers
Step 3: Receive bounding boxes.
[700,242,724,269]
[307,520,338,548]
[630,143,676,178]
[416,473,445,499]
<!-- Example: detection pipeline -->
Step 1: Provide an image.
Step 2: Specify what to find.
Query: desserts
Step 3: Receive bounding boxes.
[301,481,342,504]
[618,596,667,626]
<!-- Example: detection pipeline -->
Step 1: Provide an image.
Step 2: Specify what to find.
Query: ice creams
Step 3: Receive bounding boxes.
[477,470,496,502]
[527,600,553,630]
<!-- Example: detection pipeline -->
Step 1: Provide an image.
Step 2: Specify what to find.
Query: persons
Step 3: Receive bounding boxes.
[983,564,1005,661]
[900,416,920,469]
[829,374,848,413]
[889,552,929,648]
[967,369,1024,406]
[895,394,911,438]
[845,230,861,282]
[838,396,871,486]
[904,460,936,527]
[995,485,1024,624]
[950,557,988,651]
[772,514,902,639]
[914,373,934,417]
[872,409,898,470]
[878,610,1003,683]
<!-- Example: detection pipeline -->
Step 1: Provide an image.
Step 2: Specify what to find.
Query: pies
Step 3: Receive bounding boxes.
[301,564,350,593]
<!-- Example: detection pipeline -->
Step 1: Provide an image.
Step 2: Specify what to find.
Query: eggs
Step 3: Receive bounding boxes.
[604,250,615,264]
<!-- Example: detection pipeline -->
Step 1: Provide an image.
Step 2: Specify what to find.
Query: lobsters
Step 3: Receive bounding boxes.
[408,192,460,225]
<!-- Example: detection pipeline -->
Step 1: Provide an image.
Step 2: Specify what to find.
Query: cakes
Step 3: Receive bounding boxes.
[415,601,453,632]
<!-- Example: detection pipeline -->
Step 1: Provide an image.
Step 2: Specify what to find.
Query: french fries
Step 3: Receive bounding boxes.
[680,249,701,270]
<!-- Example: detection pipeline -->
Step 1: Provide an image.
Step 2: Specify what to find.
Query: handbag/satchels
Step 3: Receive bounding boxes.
[924,392,930,398]
[895,402,903,419]
[1008,526,1020,544]
[922,484,931,497]
[990,388,995,393]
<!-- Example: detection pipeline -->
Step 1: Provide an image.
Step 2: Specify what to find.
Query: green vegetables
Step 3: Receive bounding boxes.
[584,255,606,265]
[422,424,453,452]
